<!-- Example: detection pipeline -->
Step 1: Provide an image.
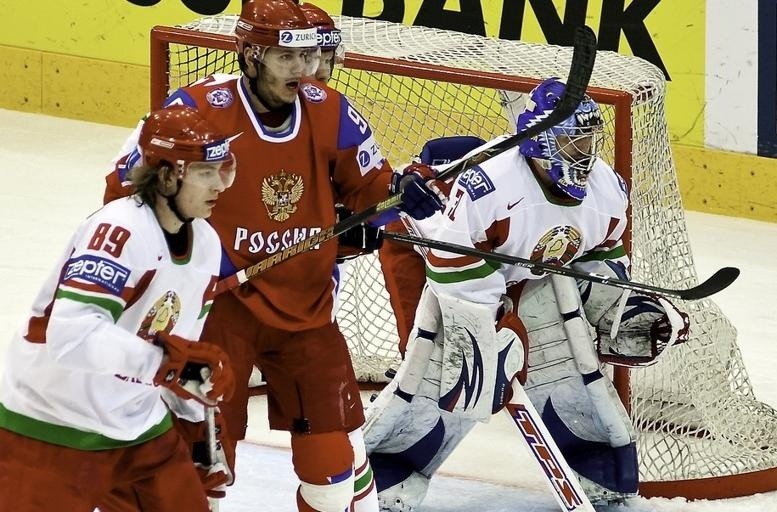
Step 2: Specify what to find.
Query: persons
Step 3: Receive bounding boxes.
[104,0,451,512]
[0,105,237,510]
[185,1,383,512]
[361,75,690,511]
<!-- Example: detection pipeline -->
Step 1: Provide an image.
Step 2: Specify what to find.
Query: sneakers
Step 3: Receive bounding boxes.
[378,473,431,509]
[574,471,640,504]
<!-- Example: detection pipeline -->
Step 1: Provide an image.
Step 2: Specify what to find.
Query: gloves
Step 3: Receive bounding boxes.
[654,311,691,346]
[154,327,236,409]
[391,172,441,218]
[192,442,229,498]
[336,210,384,257]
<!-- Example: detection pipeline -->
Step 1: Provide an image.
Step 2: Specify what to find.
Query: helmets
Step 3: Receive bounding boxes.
[517,74,606,201]
[137,107,234,176]
[233,0,318,60]
[295,0,340,54]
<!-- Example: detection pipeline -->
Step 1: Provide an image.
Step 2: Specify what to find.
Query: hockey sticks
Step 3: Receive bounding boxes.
[213,24,598,297]
[381,231,740,300]
